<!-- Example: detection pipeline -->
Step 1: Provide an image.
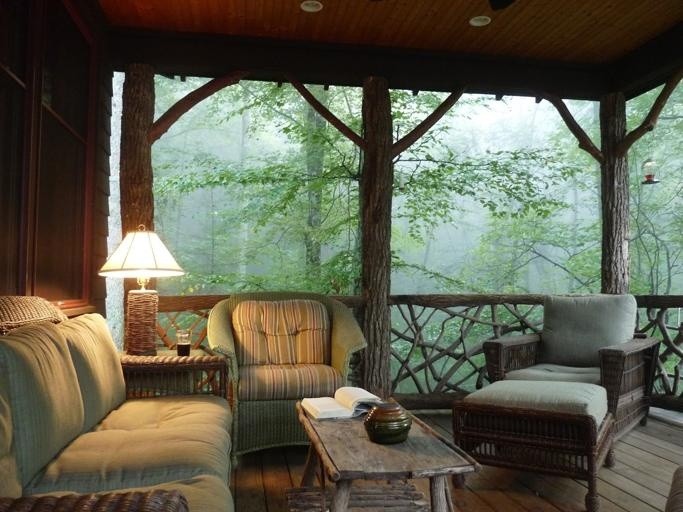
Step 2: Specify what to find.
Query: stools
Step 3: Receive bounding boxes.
[452,380,614,510]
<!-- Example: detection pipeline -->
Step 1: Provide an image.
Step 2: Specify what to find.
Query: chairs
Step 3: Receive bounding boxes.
[207,291,367,466]
[483,333,661,463]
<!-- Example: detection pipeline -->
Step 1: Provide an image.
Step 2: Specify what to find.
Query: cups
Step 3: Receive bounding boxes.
[177,330,191,357]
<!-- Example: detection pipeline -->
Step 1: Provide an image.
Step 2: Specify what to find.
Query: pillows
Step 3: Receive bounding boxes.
[539,294,638,366]
[233,300,330,365]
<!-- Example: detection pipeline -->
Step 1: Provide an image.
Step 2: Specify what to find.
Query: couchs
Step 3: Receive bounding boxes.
[0,313,235,512]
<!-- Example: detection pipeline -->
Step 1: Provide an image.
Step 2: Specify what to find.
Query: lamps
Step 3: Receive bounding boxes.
[97,224,184,355]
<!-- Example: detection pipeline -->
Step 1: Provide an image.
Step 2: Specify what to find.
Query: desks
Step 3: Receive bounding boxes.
[118,344,229,401]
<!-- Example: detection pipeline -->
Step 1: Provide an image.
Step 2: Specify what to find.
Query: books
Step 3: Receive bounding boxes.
[300,385,381,420]
